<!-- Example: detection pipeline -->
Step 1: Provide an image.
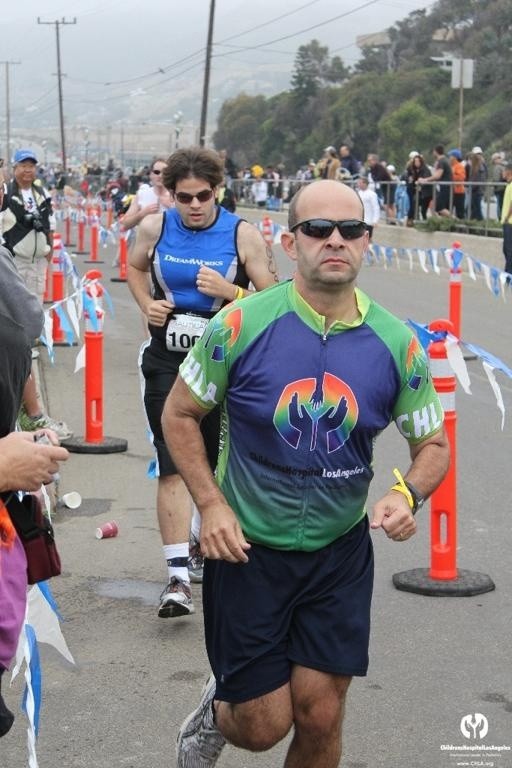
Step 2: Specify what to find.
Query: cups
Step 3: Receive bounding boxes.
[61,491,82,508]
[94,521,118,538]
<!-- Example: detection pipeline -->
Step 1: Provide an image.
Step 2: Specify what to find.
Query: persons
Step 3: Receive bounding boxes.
[36,140,512,279]
[1,146,74,437]
[126,146,283,616]
[176,179,451,768]
[0,153,70,737]
[119,158,175,340]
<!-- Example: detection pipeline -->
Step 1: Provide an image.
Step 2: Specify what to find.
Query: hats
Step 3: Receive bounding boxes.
[387,165,394,172]
[14,149,39,163]
[409,152,418,160]
[323,146,336,154]
[447,150,462,160]
[473,146,481,154]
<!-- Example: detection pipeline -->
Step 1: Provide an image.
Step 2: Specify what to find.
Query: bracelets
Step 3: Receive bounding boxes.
[392,480,425,516]
[236,285,243,300]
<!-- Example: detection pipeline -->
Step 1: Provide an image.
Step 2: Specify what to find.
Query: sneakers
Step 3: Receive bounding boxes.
[188,536,204,585]
[33,416,74,441]
[177,672,228,768]
[157,576,194,615]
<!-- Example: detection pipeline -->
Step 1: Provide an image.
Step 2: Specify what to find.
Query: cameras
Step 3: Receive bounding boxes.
[32,433,60,485]
[24,211,45,232]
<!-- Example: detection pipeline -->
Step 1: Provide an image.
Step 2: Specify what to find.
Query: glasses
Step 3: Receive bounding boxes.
[291,218,373,242]
[152,169,162,174]
[174,188,213,204]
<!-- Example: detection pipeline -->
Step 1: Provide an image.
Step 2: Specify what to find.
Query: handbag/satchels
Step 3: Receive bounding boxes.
[8,496,61,587]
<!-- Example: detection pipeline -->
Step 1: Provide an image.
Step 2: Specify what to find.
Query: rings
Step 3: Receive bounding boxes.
[197,280,204,287]
[398,532,406,540]
